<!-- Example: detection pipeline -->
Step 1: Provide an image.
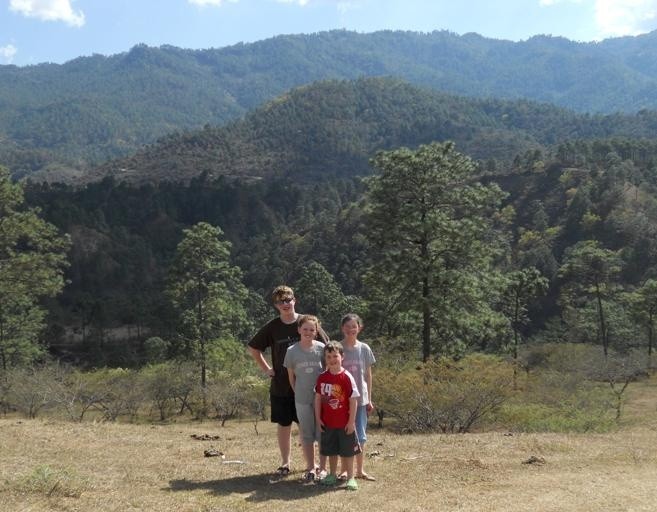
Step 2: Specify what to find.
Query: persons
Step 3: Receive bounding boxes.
[333,312,377,482]
[243,284,331,479]
[281,314,327,482]
[312,339,359,491]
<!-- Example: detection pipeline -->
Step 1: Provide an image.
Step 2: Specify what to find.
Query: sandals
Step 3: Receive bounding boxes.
[303,468,337,485]
[339,472,359,491]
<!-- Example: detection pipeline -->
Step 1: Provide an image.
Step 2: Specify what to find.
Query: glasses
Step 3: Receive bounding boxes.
[274,297,294,304]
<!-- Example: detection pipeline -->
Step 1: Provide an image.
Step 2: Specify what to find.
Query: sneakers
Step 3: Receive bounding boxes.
[270,464,290,480]
[356,472,376,481]
